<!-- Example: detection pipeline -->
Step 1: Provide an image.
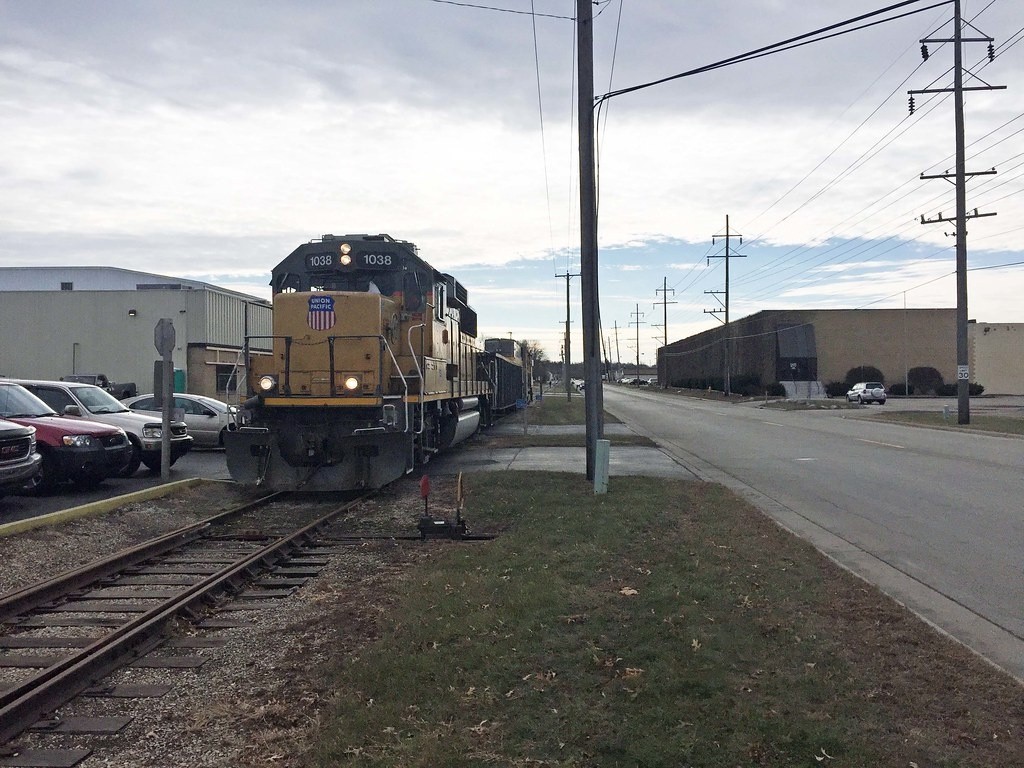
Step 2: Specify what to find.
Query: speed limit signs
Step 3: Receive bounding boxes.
[957,365,970,380]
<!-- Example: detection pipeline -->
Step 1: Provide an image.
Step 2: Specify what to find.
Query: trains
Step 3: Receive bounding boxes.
[216,234,537,495]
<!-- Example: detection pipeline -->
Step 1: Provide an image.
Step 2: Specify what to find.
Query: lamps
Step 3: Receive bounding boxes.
[129,310,136,316]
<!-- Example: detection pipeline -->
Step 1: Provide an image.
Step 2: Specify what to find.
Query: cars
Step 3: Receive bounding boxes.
[617,377,658,386]
[89,392,243,451]
[580,383,585,389]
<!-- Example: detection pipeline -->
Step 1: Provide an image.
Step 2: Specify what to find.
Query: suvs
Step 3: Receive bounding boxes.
[0,382,134,494]
[1,379,195,473]
[0,420,42,488]
[845,382,887,405]
